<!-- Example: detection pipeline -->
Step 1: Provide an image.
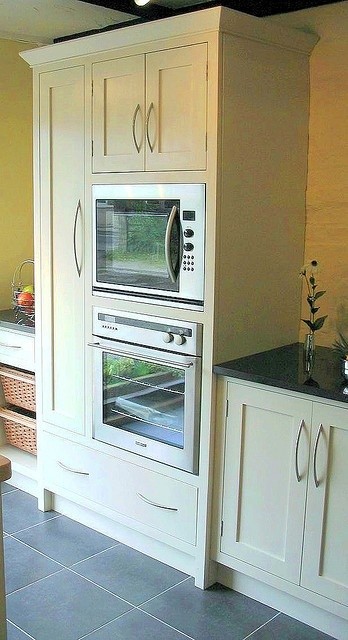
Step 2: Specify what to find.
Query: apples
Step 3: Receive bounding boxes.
[22,285,33,294]
[17,292,33,305]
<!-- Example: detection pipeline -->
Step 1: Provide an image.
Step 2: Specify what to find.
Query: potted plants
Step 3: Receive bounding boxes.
[331,333,348,395]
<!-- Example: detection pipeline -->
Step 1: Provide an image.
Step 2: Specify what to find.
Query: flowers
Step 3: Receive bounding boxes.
[299,259,328,334]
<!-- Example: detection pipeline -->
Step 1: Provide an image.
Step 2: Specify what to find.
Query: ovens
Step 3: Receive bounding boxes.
[86,306,203,475]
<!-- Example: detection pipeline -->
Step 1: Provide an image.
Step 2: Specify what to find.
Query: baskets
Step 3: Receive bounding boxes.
[1,405,37,454]
[0,366,36,412]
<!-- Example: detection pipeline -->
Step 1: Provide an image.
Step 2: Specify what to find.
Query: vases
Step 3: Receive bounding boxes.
[303,335,315,373]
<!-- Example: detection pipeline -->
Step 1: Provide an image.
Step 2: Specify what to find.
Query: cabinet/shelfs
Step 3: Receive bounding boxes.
[213,373,348,640]
[37,431,213,591]
[0,328,38,498]
[88,7,221,176]
[19,36,85,432]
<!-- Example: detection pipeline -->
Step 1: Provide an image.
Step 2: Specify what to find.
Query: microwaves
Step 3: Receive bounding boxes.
[92,183,206,312]
